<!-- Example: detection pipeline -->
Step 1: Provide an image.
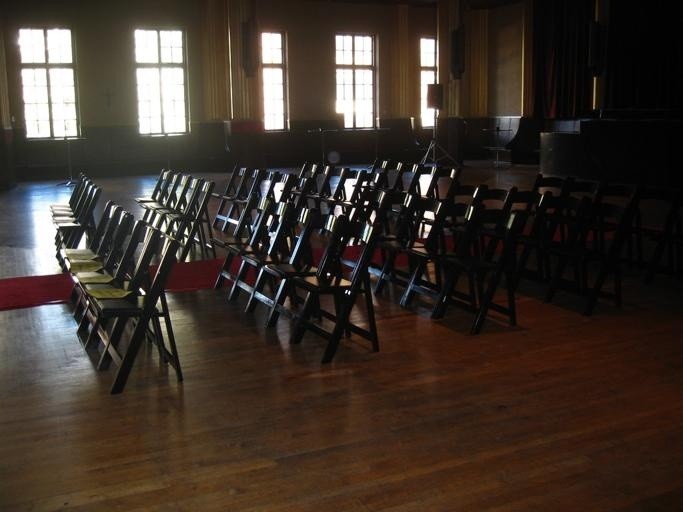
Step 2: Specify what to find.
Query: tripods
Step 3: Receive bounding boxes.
[419,128,460,169]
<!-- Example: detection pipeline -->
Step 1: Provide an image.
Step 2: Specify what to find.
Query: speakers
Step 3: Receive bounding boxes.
[428,83,443,109]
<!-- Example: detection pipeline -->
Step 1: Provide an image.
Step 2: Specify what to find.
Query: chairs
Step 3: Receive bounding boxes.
[53,169,103,261]
[368,158,442,243]
[134,168,215,260]
[57,200,184,394]
[317,190,518,332]
[443,175,649,314]
[211,196,379,363]
[299,160,377,246]
[212,162,311,240]
